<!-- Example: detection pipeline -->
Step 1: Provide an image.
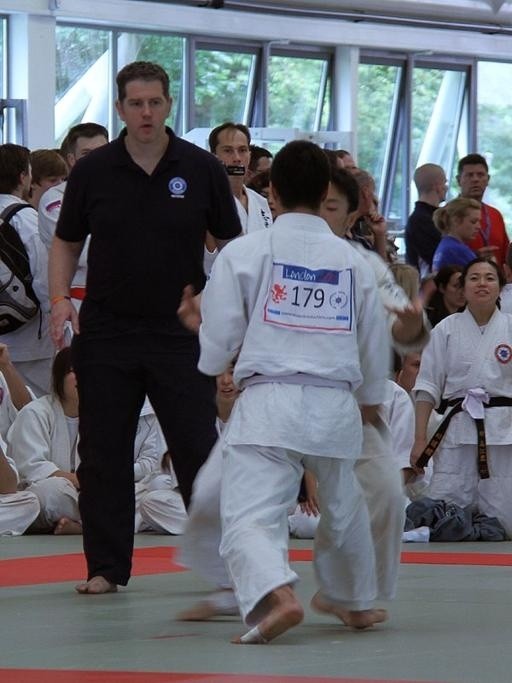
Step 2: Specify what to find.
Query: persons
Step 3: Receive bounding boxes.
[201,139,428,643]
[411,259,512,540]
[405,163,448,273]
[456,153,509,277]
[0,143,72,392]
[6,346,143,537]
[140,356,239,535]
[429,264,464,328]
[51,62,244,595]
[498,241,512,314]
[432,198,480,310]
[244,146,274,219]
[337,149,390,263]
[0,345,36,440]
[1,386,40,534]
[289,347,415,537]
[203,123,272,277]
[23,150,69,211]
[177,169,404,621]
[39,123,108,314]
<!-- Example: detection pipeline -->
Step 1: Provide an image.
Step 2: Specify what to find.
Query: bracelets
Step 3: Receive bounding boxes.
[50,295,71,304]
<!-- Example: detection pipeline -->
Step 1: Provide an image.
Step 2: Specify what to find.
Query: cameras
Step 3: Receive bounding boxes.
[227,165,244,176]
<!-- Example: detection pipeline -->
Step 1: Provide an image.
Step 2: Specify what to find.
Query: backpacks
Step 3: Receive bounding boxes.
[0,202,42,334]
